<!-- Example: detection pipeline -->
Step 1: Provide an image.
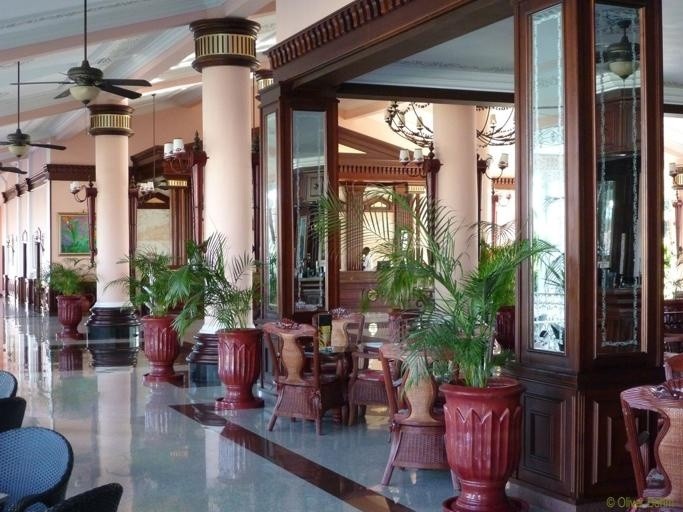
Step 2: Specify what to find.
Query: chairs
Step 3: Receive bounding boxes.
[348,308,420,425]
[0,427,74,512]
[0,397,26,431]
[55,483,124,512]
[262,319,345,435]
[649,398,683,510]
[379,343,460,489]
[0,371,17,397]
[312,308,365,378]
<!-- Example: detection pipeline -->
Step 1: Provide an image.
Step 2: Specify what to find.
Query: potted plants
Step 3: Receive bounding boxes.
[311,190,556,512]
[50,263,84,340]
[103,250,195,385]
[166,232,265,409]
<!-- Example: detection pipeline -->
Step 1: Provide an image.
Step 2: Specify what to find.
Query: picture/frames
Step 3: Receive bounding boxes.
[58,212,90,256]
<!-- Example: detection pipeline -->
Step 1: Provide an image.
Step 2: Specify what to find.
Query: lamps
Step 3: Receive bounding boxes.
[70,86,99,136]
[151,188,160,193]
[399,142,444,265]
[164,130,207,245]
[129,175,155,259]
[70,176,97,267]
[8,145,27,157]
[609,62,640,109]
[477,153,509,262]
[384,100,515,148]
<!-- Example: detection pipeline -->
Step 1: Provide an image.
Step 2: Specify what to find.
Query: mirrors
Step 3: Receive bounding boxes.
[581,0,663,371]
[515,0,580,377]
[253,80,286,328]
[287,95,340,324]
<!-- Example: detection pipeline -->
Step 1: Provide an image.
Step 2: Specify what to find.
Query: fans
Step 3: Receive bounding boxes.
[129,94,168,190]
[0,62,66,150]
[10,0,151,99]
[592,19,639,64]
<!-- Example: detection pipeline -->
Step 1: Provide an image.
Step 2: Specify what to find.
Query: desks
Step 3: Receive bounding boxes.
[620,386,683,512]
